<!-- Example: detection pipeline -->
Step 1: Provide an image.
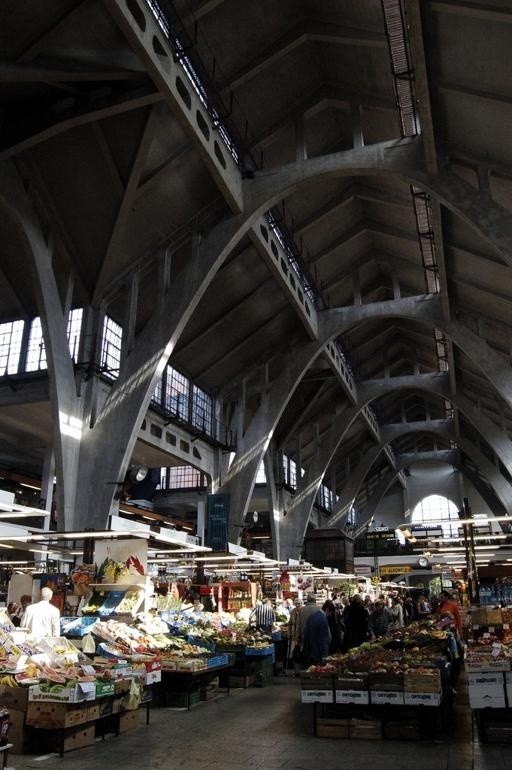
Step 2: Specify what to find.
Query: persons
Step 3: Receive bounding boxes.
[11,594,32,627]
[438,590,463,661]
[18,586,61,638]
[248,598,276,635]
[6,601,18,621]
[282,589,430,678]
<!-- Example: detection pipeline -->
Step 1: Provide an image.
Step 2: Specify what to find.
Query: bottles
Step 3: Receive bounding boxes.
[0,705,10,748]
[477,584,512,605]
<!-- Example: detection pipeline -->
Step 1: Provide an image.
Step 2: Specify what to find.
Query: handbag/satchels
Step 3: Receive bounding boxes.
[292,644,303,664]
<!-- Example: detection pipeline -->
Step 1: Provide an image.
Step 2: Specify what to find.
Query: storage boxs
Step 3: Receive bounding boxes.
[0,606,512,757]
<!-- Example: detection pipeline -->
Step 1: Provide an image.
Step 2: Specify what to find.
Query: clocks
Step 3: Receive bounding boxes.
[418,557,429,568]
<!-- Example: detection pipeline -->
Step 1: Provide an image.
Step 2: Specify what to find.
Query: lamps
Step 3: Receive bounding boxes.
[129,466,150,484]
[246,512,258,524]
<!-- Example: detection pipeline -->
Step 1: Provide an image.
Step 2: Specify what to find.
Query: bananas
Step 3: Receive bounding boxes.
[1,674,19,687]
[433,631,448,638]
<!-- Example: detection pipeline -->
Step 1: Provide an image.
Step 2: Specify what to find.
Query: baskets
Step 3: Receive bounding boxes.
[245,644,274,655]
[207,654,229,668]
[167,689,199,707]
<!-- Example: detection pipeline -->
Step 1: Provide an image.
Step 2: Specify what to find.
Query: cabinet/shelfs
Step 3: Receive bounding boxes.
[157,561,372,612]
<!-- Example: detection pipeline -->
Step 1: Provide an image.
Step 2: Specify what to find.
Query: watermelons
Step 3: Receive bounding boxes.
[39,674,80,694]
